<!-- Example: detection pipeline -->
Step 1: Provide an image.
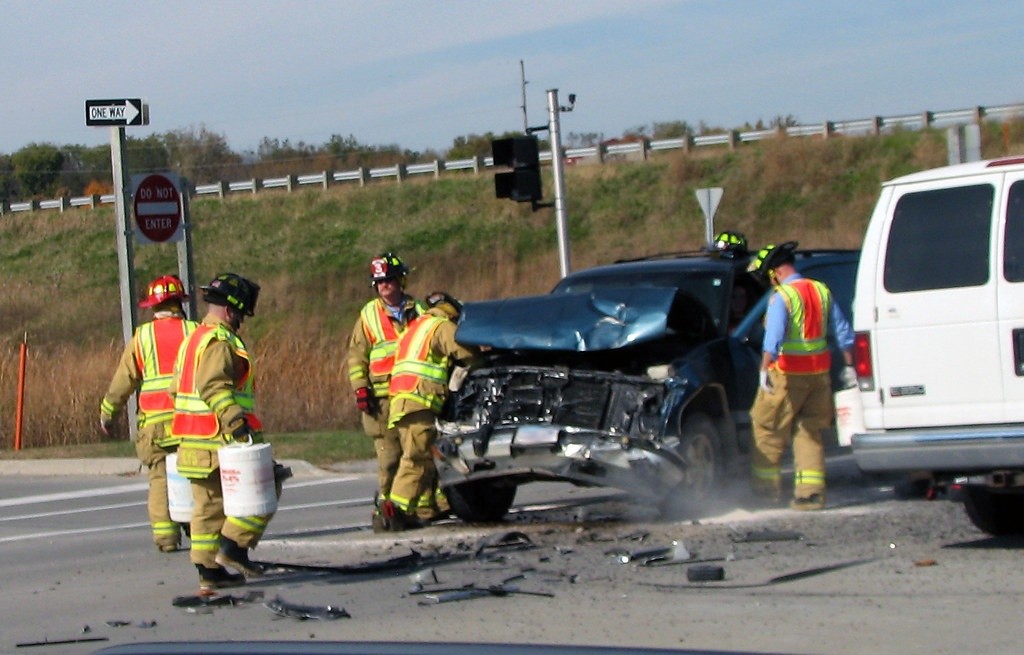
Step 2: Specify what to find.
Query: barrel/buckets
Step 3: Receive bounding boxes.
[165,451,195,523]
[217,434,277,518]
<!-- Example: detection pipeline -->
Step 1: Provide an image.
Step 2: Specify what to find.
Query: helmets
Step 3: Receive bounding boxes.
[139,274,192,307]
[199,273,260,317]
[426,291,463,321]
[708,230,747,251]
[746,241,797,287]
[370,252,408,280]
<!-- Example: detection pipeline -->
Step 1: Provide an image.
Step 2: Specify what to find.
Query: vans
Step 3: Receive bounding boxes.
[851,156,1024,539]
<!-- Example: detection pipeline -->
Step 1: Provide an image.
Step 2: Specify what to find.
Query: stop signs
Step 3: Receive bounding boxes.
[131,172,185,244]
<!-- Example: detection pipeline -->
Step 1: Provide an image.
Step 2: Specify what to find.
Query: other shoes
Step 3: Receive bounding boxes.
[389,508,422,530]
[445,509,453,515]
[373,514,390,533]
[752,489,784,504]
[429,512,449,521]
[195,564,246,587]
[161,544,176,552]
[790,494,825,510]
[217,541,263,576]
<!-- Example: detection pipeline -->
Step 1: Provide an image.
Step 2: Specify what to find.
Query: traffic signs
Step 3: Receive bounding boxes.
[84,97,143,127]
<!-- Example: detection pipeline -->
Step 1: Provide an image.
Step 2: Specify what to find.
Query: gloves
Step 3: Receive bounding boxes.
[841,366,857,387]
[355,387,374,414]
[101,411,112,434]
[760,371,775,395]
[229,413,256,442]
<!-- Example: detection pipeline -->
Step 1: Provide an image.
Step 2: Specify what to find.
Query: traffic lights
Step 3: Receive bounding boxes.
[492,135,545,203]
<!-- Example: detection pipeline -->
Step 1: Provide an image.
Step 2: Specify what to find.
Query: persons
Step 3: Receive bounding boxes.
[99,275,202,553]
[746,245,856,511]
[370,290,482,535]
[169,273,284,590]
[347,252,453,525]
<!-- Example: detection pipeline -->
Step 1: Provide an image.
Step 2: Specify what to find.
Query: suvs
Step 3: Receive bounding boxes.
[428,242,864,524]
